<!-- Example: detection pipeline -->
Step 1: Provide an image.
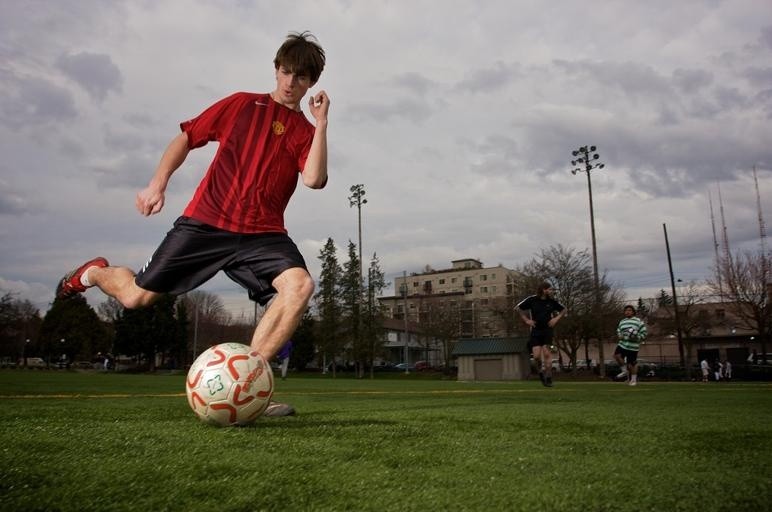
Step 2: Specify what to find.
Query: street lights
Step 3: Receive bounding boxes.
[672,280,685,367]
[348,184,367,327]
[572,146,607,377]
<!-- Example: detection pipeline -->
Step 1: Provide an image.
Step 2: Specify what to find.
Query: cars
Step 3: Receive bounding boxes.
[395,361,427,370]
[530,354,657,372]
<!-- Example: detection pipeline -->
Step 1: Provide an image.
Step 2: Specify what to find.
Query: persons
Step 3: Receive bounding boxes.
[277,339,292,381]
[513,280,566,388]
[700,357,733,382]
[613,304,648,387]
[52,29,332,418]
[103,356,109,373]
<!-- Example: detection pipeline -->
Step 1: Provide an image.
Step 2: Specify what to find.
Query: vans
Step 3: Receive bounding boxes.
[19,357,47,367]
[748,353,772,364]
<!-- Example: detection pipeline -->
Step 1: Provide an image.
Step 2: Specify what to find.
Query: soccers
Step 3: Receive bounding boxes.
[186,342,275,425]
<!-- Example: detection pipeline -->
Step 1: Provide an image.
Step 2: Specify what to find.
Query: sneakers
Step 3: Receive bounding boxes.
[55,256,109,300]
[617,370,637,386]
[265,400,293,416]
